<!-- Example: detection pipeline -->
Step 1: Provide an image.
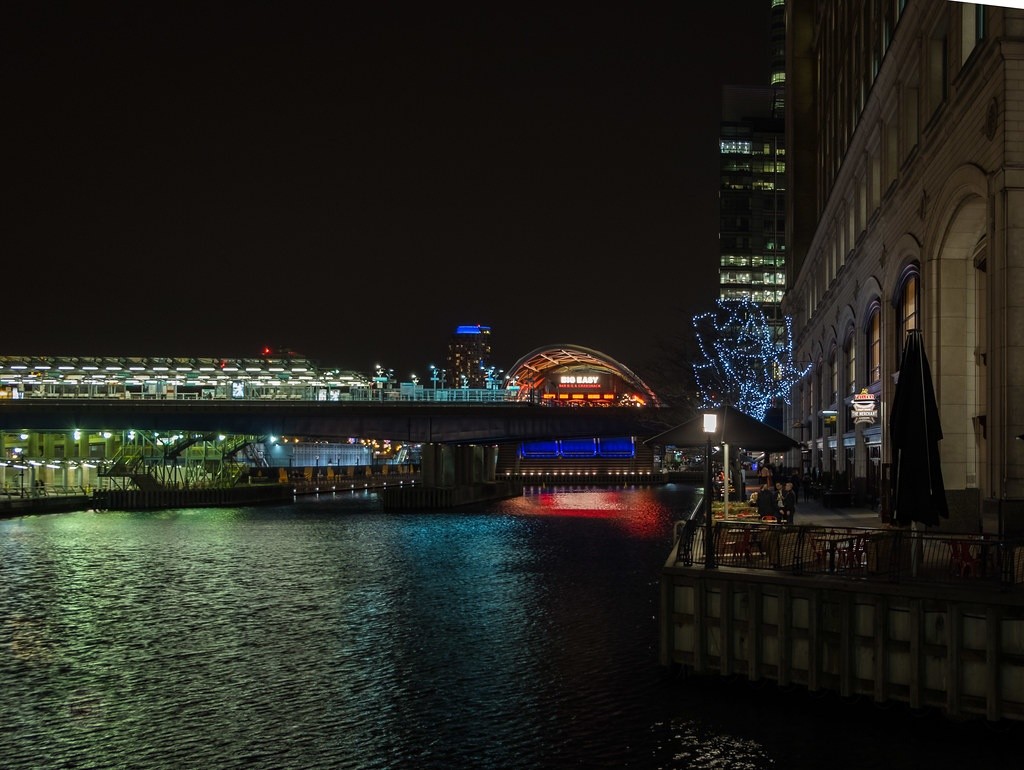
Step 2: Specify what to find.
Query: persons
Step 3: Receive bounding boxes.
[781,483,796,526]
[802,473,812,504]
[774,483,787,526]
[755,484,774,516]
[790,470,800,504]
[757,462,792,485]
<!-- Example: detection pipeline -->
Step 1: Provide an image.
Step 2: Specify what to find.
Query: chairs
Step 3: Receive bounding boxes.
[213,395,227,399]
[31,392,121,400]
[748,531,766,557]
[841,532,866,569]
[806,535,830,571]
[259,394,303,400]
[720,536,736,560]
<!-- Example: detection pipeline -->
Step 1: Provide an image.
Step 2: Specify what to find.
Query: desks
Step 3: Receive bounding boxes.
[727,529,763,562]
[941,540,991,578]
[131,392,199,400]
[813,530,871,573]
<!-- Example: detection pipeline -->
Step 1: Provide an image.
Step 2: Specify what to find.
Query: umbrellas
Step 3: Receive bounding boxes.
[642,405,803,521]
[703,446,756,483]
[888,330,949,577]
